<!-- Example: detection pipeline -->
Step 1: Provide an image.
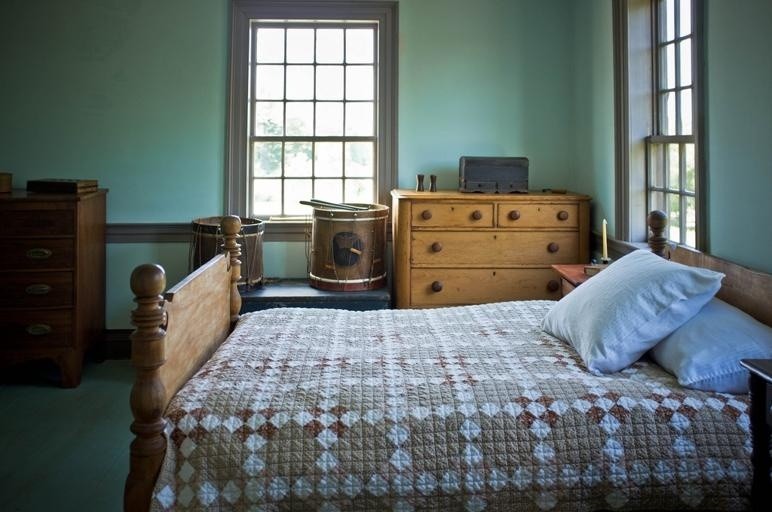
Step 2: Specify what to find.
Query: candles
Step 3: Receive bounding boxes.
[601,218,608,259]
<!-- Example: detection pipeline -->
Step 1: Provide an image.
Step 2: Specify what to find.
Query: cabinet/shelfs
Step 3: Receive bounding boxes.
[0,184,110,390]
[387,184,594,310]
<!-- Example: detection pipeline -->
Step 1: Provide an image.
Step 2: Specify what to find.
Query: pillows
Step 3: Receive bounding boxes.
[647,295,771,393]
[539,247,726,377]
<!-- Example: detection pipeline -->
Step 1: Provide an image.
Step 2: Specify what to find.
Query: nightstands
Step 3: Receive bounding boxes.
[551,262,610,299]
[735,355,772,512]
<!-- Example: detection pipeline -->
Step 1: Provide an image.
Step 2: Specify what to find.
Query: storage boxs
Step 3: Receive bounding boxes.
[238,276,391,315]
[458,155,530,195]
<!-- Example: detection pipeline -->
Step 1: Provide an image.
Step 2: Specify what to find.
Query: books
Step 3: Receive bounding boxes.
[25,178,98,195]
[583,263,607,276]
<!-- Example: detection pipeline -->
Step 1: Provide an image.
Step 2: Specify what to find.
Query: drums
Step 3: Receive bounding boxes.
[189,215,264,295]
[309,203,390,293]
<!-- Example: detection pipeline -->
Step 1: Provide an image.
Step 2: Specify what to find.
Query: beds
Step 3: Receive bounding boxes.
[121,209,771,512]
